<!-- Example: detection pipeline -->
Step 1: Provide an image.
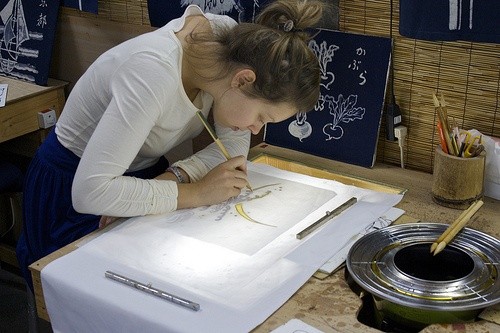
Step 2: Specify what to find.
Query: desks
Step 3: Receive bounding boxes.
[0,75,69,268]
[28,142,500,333]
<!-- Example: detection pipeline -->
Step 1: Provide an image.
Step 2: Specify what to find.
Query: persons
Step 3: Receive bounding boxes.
[15,0,339,295]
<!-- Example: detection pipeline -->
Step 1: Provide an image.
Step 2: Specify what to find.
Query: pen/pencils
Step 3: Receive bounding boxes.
[432,93,484,158]
[431,199,483,255]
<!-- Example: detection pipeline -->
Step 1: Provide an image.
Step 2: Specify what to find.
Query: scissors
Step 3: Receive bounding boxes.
[459,132,482,157]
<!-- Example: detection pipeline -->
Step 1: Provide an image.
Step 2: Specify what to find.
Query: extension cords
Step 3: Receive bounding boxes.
[387,95,402,141]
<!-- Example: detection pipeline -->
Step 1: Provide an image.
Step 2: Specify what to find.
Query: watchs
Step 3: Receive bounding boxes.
[166,167,185,183]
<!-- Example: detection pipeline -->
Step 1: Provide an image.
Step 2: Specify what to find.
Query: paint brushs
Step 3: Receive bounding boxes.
[197,111,253,192]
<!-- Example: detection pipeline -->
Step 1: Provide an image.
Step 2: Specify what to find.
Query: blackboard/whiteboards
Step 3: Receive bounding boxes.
[0,0,62,87]
[263,27,394,172]
